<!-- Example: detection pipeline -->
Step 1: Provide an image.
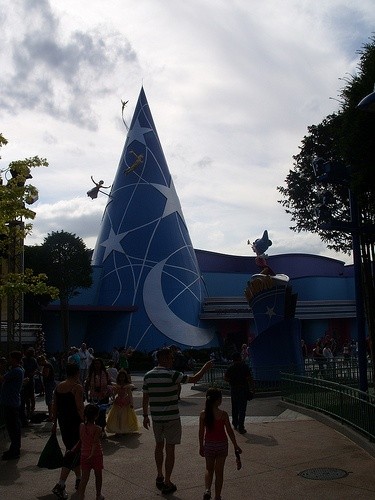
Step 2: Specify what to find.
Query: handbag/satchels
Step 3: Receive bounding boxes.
[37,431,65,469]
[247,390,254,401]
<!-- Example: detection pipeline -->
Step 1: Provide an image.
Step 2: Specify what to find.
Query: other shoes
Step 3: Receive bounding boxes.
[203,490,211,500]
[240,429,247,434]
[27,418,31,423]
[162,482,176,492]
[52,483,68,500]
[1,449,21,461]
[74,479,81,489]
[156,477,164,485]
[96,495,104,500]
[234,425,238,430]
[43,417,53,422]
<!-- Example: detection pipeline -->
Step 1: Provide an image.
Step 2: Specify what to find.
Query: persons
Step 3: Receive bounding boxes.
[0,351,25,460]
[224,353,255,435]
[106,368,138,438]
[0,335,375,426]
[142,349,215,493]
[68,404,105,500]
[52,364,84,499]
[82,358,112,440]
[199,389,242,500]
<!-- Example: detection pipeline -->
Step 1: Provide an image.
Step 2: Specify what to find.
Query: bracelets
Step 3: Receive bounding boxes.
[143,414,148,417]
